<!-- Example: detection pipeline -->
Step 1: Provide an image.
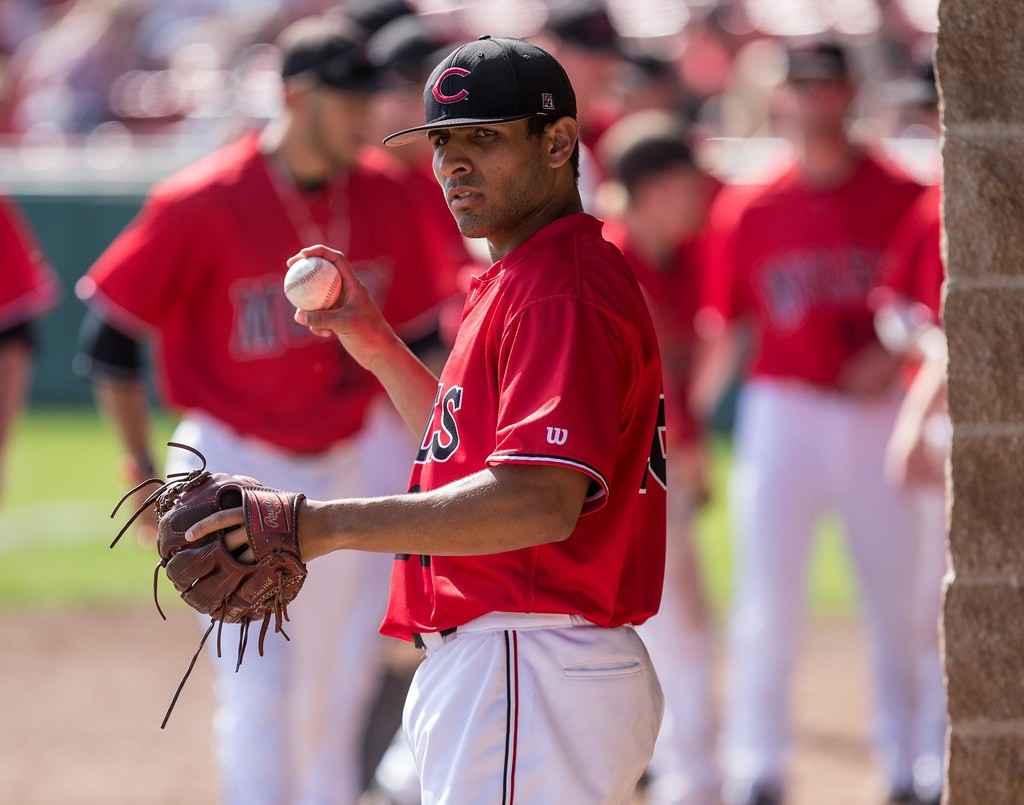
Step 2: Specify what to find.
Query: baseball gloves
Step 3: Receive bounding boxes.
[157,472,308,623]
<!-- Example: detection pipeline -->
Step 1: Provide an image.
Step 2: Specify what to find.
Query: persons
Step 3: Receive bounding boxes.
[76,0,482,804]
[111,34,665,804]
[528,0,724,804]
[685,38,950,805]
[1,197,64,445]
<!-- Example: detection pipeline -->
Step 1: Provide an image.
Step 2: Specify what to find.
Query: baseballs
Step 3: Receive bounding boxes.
[283,257,342,312]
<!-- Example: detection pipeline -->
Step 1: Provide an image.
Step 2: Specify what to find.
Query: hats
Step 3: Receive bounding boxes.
[380,34,578,151]
[785,43,856,89]
[619,137,692,190]
[279,14,381,102]
